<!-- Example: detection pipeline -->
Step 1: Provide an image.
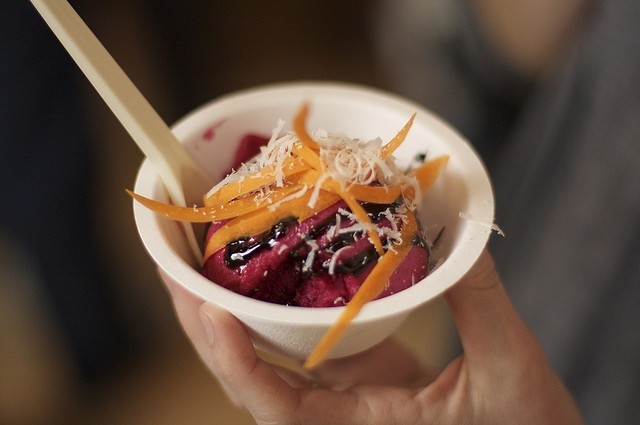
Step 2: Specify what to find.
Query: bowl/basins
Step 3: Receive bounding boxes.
[133,80,496,364]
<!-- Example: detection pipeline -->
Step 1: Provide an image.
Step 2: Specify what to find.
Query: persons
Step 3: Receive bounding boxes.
[156,1,640,425]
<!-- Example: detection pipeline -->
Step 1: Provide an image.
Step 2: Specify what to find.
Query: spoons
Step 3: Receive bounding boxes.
[31,0,215,265]
